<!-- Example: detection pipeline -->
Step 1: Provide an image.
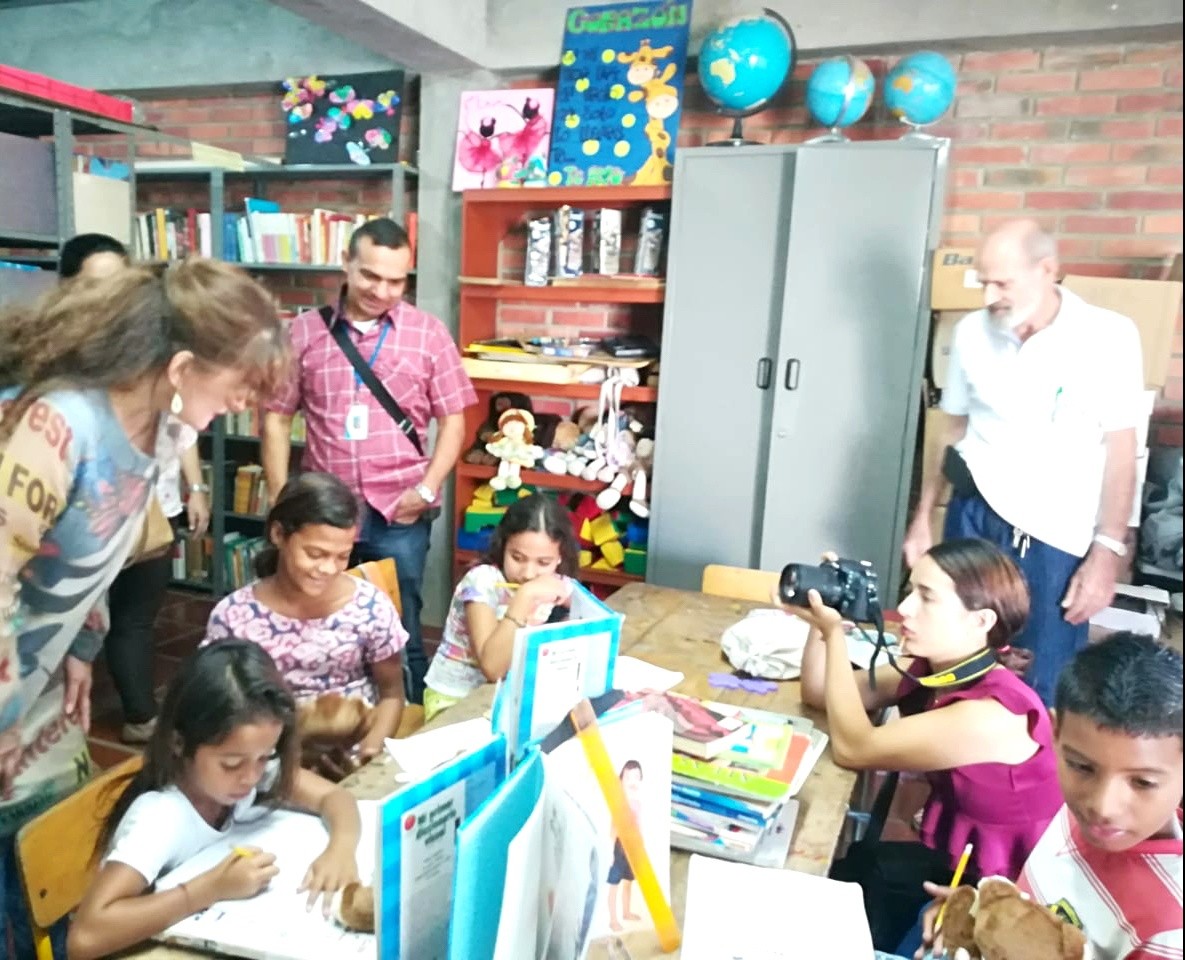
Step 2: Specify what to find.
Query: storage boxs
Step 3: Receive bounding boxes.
[632,205,668,276]
[931,310,970,389]
[919,377,954,506]
[553,203,584,276]
[931,244,988,310]
[523,216,551,287]
[1058,272,1183,384]
[589,207,623,275]
[1089,581,1172,640]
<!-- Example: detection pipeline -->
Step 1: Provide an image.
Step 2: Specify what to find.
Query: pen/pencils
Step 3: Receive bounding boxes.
[229,845,254,857]
[495,583,521,587]
[934,843,972,936]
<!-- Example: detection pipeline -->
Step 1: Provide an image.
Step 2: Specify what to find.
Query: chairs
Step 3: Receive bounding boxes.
[345,557,417,706]
[12,753,147,960]
[700,563,781,604]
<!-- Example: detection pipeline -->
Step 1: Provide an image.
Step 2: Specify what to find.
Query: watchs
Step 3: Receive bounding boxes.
[415,483,436,505]
[1092,533,1127,557]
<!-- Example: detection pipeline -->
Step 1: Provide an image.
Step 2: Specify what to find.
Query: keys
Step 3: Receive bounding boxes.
[1010,527,1032,559]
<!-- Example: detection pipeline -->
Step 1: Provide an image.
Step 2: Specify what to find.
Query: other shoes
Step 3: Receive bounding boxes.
[122,716,159,742]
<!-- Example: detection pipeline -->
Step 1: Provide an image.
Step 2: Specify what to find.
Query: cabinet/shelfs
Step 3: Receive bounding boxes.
[646,138,951,609]
[135,162,419,603]
[0,88,159,273]
[453,184,673,600]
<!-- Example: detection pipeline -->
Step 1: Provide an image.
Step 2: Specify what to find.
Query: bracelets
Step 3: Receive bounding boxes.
[503,613,527,629]
[185,483,210,494]
[178,882,193,917]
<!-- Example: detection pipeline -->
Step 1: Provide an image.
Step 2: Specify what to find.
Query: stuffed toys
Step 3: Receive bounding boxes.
[483,400,654,520]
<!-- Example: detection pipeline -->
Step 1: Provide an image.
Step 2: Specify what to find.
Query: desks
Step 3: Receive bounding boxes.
[114,581,911,960]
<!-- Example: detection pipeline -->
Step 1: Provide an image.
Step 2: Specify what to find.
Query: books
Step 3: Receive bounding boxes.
[162,302,309,590]
[361,576,831,960]
[131,194,419,276]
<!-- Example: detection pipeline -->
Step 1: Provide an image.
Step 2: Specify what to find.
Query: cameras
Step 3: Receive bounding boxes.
[779,558,879,621]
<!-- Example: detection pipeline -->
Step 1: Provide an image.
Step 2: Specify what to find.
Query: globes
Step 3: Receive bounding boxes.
[883,50,956,140]
[804,54,875,143]
[698,8,797,146]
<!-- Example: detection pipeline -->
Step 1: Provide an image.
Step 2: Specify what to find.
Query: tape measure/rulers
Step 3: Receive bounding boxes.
[570,697,680,953]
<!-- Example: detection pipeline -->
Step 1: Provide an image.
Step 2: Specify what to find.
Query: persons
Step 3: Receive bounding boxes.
[422,491,580,730]
[256,217,480,705]
[195,470,426,783]
[65,636,361,960]
[52,232,212,743]
[909,630,1183,960]
[800,535,1069,884]
[898,216,1146,709]
[0,257,299,960]
